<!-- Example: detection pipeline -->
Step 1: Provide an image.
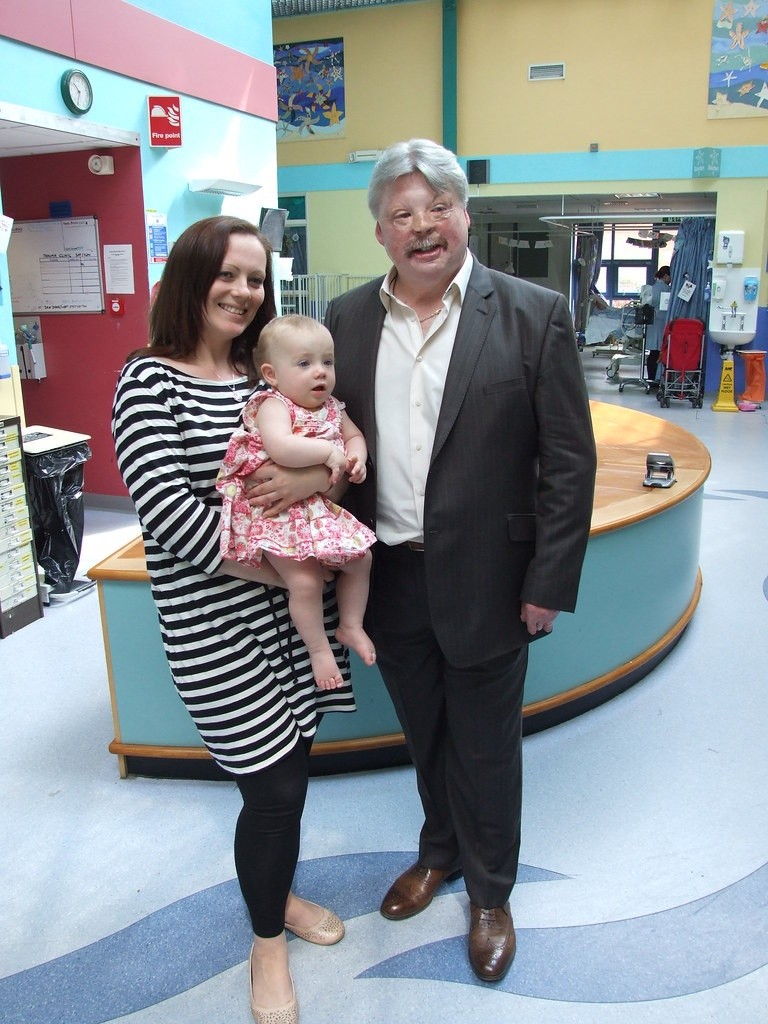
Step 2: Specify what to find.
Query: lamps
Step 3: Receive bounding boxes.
[348,149,386,164]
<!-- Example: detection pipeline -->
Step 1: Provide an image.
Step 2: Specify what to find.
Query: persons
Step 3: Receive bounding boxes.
[111,215,345,1024]
[646,265,671,379]
[326,138,598,981]
[215,312,376,690]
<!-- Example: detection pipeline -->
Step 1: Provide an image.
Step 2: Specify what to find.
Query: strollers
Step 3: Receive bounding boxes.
[657,317,707,409]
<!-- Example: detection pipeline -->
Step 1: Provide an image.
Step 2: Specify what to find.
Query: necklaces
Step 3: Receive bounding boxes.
[197,355,243,403]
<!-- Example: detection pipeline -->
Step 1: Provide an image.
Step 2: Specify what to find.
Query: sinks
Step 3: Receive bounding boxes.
[709,330,756,345]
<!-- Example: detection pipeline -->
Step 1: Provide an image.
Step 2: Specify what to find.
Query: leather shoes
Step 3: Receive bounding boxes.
[379,856,467,919]
[469,899,515,982]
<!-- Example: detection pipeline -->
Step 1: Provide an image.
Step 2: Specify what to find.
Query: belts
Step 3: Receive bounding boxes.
[401,540,427,553]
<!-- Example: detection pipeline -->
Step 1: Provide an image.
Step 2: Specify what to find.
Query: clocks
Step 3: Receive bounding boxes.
[61,68,94,114]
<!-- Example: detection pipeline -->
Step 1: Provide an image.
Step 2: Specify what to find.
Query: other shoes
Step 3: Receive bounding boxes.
[282,889,344,947]
[248,925,298,1024]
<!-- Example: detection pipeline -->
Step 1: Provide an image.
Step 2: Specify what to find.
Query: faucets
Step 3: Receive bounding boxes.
[739,315,745,331]
[721,316,726,330]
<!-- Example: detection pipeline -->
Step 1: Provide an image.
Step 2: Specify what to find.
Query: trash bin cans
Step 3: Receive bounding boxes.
[21,424,92,593]
[736,349,767,402]
